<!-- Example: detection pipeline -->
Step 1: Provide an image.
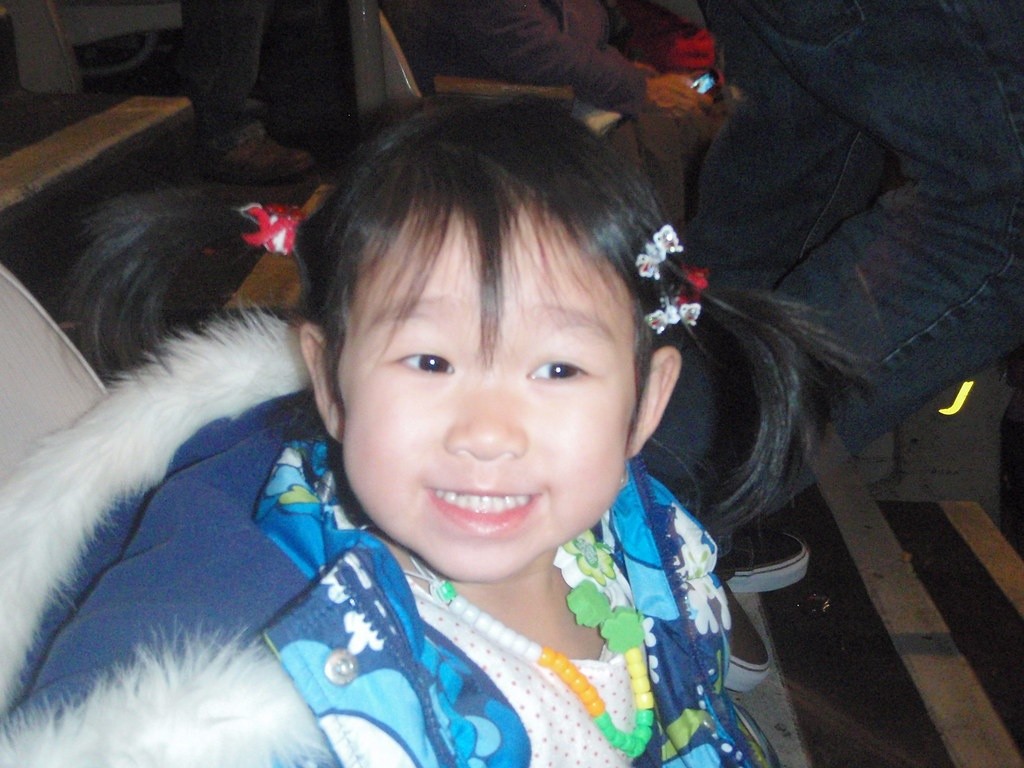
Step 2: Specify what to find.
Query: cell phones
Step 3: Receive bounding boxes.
[692,67,724,97]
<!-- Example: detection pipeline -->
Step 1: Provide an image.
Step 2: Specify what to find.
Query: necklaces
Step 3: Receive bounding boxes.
[360,517,653,759]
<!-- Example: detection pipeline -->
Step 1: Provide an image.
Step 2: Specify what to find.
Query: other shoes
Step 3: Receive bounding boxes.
[725,519,810,593]
[190,132,315,178]
[713,568,769,693]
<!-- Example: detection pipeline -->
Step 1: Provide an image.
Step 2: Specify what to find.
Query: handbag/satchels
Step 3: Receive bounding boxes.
[611,0,724,100]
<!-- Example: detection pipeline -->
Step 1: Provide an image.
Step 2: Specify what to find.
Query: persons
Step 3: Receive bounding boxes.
[24,88,782,768]
[186,0,1024,697]
